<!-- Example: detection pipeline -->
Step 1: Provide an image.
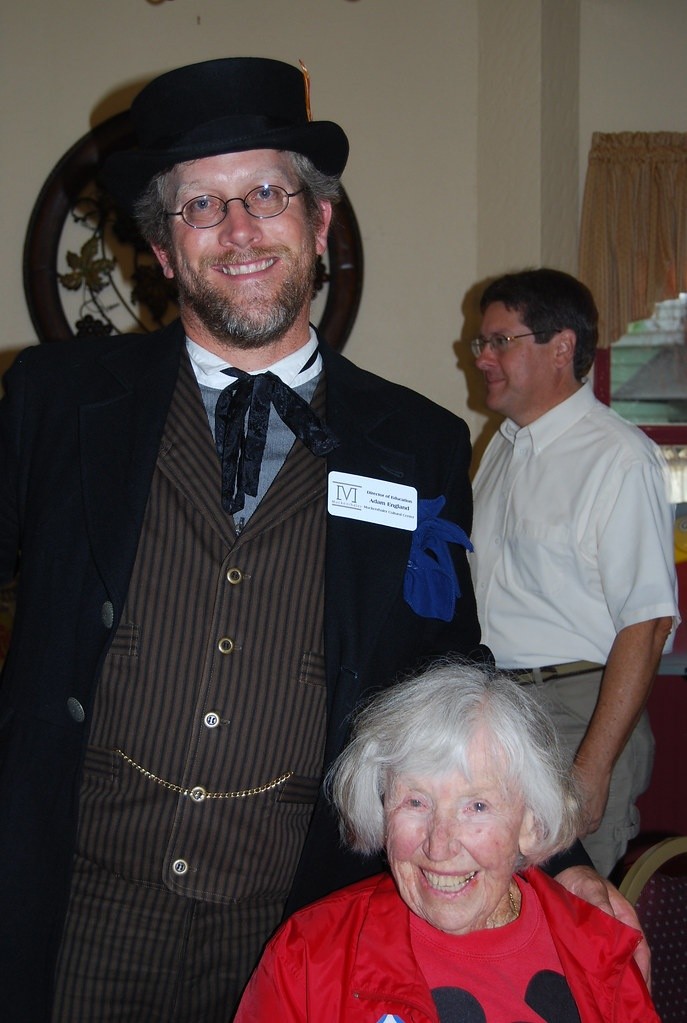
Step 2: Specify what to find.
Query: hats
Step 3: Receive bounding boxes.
[120,58,350,196]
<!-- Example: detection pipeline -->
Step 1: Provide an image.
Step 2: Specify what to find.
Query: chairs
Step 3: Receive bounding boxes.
[619,836,687,1023]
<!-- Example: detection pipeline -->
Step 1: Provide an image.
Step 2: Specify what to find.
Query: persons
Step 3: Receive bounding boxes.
[235,664,659,1023]
[453,268,681,887]
[1,55,651,1023]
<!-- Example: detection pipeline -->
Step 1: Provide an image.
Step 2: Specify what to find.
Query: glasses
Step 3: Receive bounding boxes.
[166,185,305,228]
[469,329,562,359]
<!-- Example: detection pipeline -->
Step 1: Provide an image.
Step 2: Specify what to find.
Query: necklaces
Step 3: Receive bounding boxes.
[508,885,524,923]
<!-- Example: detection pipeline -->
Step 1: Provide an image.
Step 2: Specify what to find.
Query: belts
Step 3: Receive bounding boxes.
[499,659,605,686]
